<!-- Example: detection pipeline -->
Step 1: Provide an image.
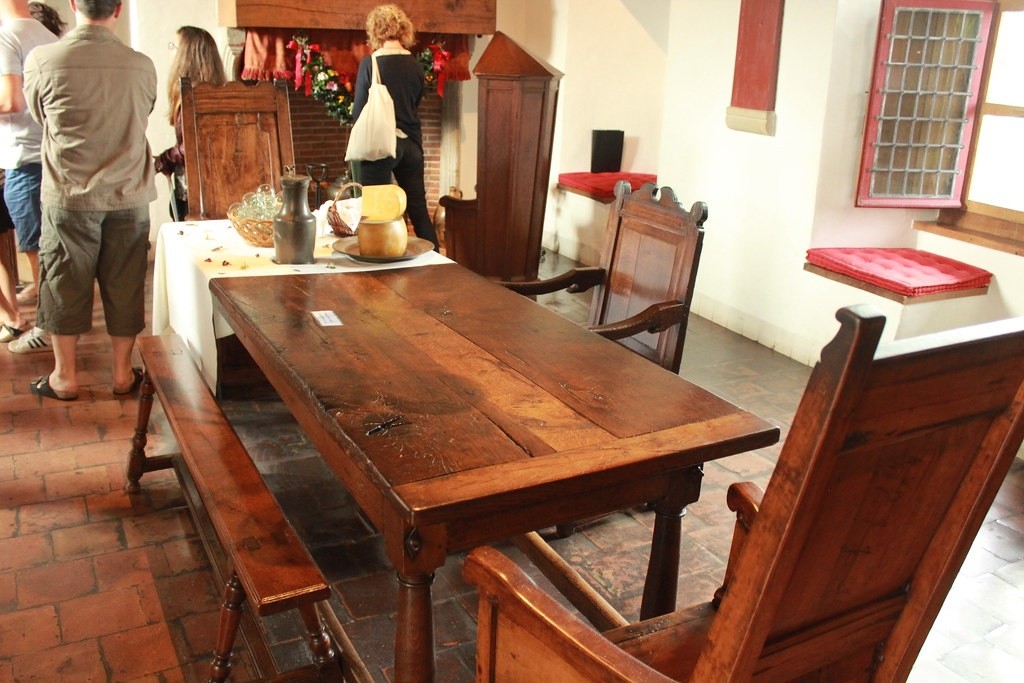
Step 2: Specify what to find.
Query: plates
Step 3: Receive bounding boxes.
[332,235,435,263]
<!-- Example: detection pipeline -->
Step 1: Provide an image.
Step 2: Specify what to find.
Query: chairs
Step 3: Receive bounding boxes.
[492,179,709,540]
[462,301,1024,683]
[179,73,296,221]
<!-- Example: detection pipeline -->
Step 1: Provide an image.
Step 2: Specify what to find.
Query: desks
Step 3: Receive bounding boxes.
[150,204,781,683]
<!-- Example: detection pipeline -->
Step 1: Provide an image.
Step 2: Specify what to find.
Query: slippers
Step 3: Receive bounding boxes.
[1,313,29,341]
[113,366,143,396]
[31,368,79,400]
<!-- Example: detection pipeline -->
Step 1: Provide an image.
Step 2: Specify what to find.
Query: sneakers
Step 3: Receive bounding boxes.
[7,323,80,354]
[16,283,39,305]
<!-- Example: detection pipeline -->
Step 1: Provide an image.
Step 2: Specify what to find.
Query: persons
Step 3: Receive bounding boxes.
[0,0,63,352]
[345,4,442,254]
[153,25,228,225]
[23,0,157,401]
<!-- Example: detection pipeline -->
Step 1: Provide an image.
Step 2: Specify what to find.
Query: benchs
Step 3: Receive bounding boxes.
[125,334,345,683]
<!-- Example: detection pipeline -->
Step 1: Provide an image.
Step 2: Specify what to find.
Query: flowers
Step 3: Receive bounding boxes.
[291,34,445,126]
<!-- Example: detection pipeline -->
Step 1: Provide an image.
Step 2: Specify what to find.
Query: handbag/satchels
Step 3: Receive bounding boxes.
[344,54,396,163]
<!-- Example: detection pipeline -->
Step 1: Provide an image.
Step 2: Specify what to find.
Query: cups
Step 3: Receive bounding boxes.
[227,184,284,222]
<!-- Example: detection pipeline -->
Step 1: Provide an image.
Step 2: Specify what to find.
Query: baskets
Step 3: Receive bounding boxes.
[228,184,284,247]
[327,182,364,236]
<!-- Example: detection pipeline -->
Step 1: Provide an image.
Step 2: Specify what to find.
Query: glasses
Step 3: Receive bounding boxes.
[166,42,178,51]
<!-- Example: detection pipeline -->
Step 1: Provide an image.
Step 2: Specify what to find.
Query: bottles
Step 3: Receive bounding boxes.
[274,173,318,265]
